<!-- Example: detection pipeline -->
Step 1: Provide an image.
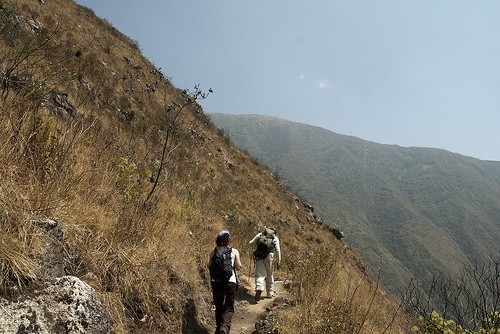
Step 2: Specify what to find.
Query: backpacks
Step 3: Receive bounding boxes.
[254,229,274,261]
[209,247,233,283]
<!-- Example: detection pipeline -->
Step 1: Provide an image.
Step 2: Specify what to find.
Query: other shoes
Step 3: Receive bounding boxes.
[254,290,262,301]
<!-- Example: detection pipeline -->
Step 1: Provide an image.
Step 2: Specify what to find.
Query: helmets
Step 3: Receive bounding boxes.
[271,228,276,235]
[216,231,229,246]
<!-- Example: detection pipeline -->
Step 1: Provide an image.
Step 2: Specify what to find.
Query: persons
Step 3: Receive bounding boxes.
[248,228,282,302]
[206,230,242,334]
